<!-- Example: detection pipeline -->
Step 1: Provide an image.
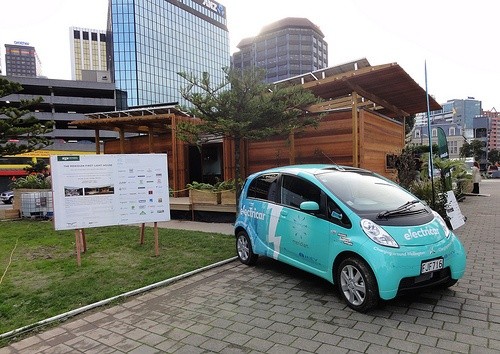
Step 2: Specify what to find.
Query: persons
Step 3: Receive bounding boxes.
[471,162,481,193]
[36,168,51,185]
[487,161,500,175]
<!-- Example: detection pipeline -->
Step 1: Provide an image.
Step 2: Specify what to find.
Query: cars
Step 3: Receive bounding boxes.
[0,190,15,204]
[233,163,467,314]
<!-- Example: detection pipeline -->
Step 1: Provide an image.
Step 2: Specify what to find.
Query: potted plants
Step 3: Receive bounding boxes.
[219,178,243,205]
[187,181,220,205]
